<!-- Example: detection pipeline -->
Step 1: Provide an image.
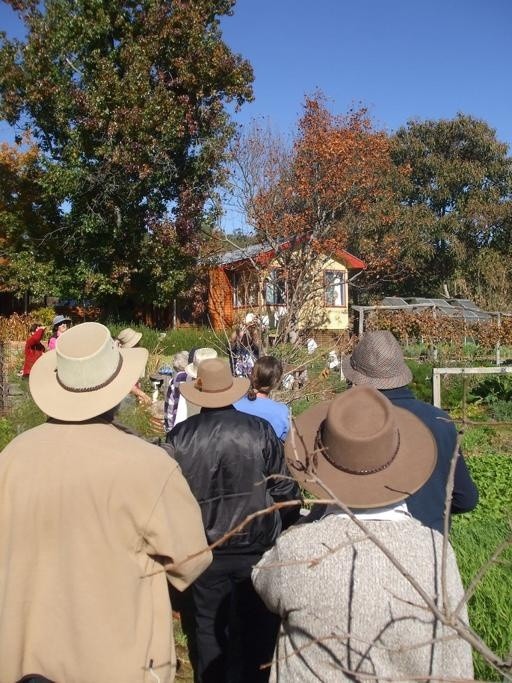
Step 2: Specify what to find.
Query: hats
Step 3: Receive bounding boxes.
[29,321,149,422]
[179,348,251,409]
[53,315,71,325]
[284,330,437,509]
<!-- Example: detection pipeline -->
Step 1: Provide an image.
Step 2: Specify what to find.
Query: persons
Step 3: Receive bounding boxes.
[166,359,301,683]
[23,315,290,443]
[251,386,477,683]
[0,322,214,683]
[294,330,479,534]
